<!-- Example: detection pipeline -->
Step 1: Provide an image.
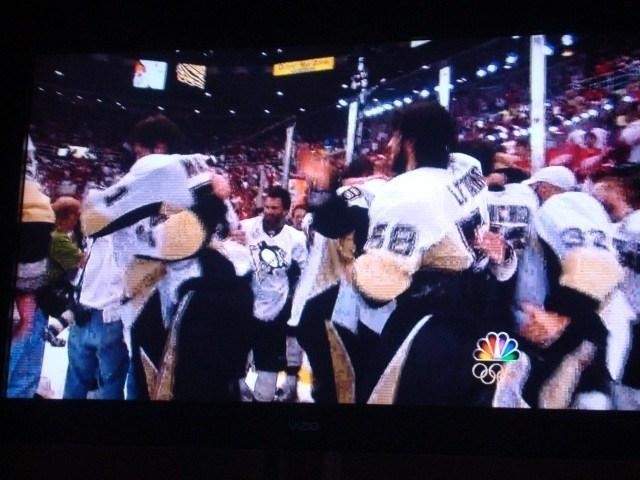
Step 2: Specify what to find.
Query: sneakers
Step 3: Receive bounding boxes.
[44,328,66,347]
[273,384,297,402]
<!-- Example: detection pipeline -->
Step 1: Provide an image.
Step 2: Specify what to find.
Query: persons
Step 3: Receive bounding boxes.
[7,56,640,409]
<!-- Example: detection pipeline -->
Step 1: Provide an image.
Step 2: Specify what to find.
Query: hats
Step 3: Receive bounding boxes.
[520,165,578,189]
